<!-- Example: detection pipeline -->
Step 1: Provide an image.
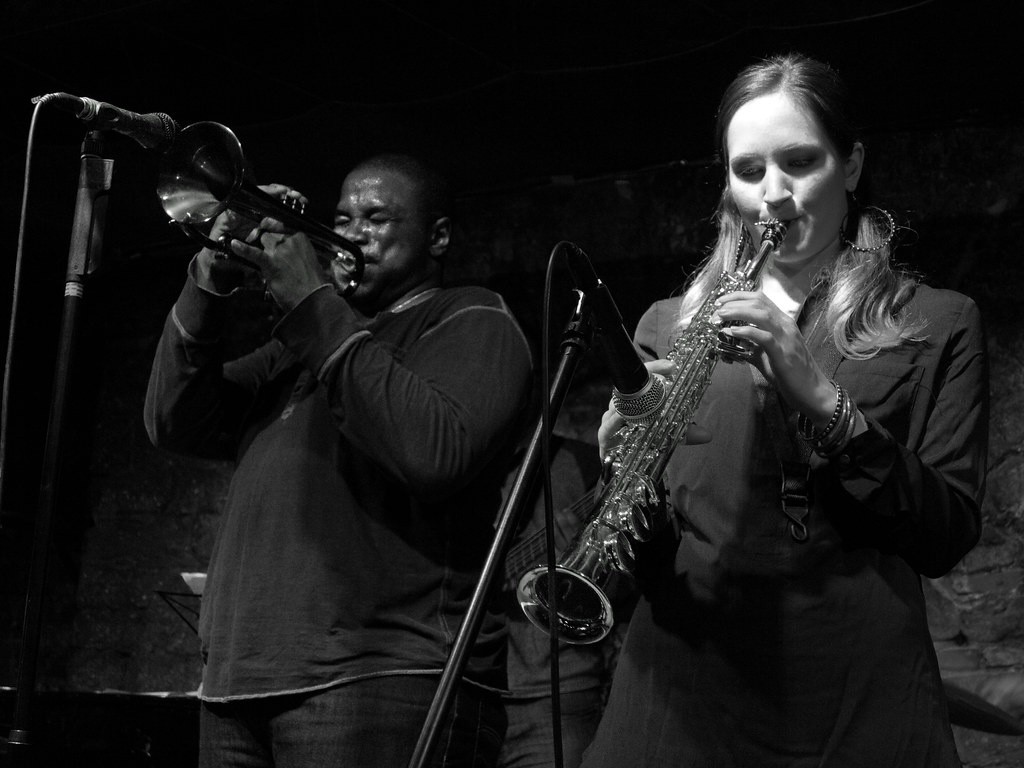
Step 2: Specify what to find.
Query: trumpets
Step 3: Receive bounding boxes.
[154,120,366,307]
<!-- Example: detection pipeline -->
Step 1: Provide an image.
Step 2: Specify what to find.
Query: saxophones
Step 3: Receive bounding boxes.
[515,217,787,648]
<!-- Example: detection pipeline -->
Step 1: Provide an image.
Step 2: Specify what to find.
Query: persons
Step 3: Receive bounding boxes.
[581,52,991,768]
[143,150,532,768]
[932,326,1024,646]
[491,304,614,768]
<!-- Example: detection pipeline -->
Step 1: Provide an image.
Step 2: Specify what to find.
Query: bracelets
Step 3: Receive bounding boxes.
[796,379,857,460]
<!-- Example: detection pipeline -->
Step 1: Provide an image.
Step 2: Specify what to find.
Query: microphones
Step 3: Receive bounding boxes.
[562,242,666,424]
[40,91,178,154]
